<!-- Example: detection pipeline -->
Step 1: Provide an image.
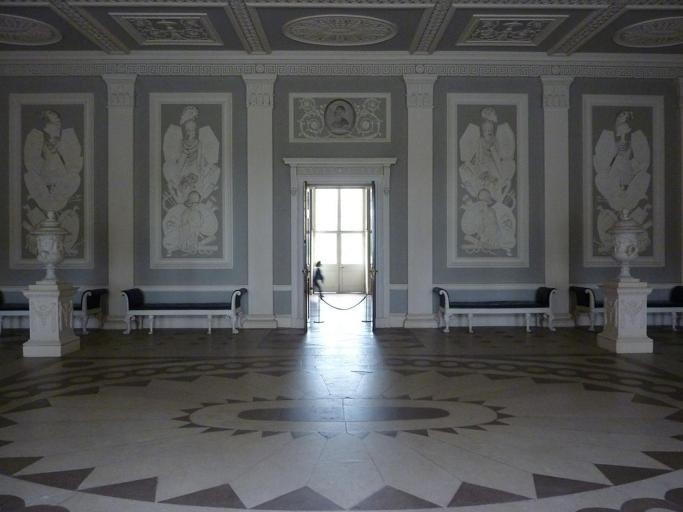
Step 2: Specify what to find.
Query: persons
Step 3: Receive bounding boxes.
[313,261,325,298]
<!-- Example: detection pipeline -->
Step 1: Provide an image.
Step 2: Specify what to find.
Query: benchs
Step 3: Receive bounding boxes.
[432,286,683,334]
[0,287,250,336]
